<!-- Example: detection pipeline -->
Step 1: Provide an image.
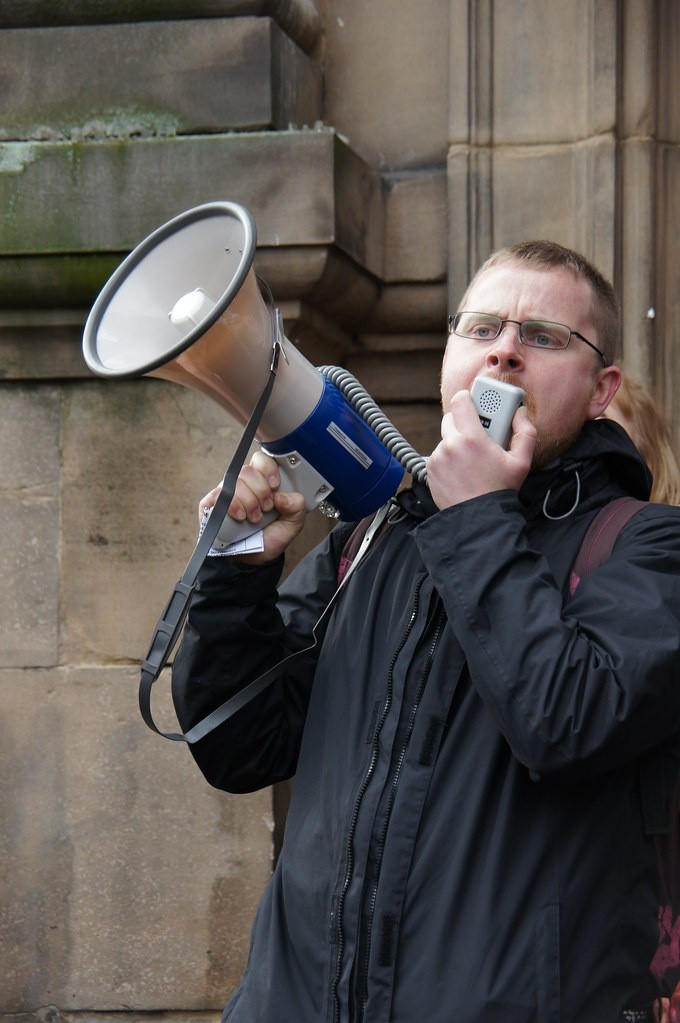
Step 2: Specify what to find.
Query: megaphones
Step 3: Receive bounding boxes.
[81,201,405,549]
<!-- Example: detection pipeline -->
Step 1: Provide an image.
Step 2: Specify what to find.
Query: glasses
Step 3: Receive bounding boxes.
[448,311,609,370]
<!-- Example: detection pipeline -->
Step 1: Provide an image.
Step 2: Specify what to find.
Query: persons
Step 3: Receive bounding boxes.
[171,238,680,1023]
